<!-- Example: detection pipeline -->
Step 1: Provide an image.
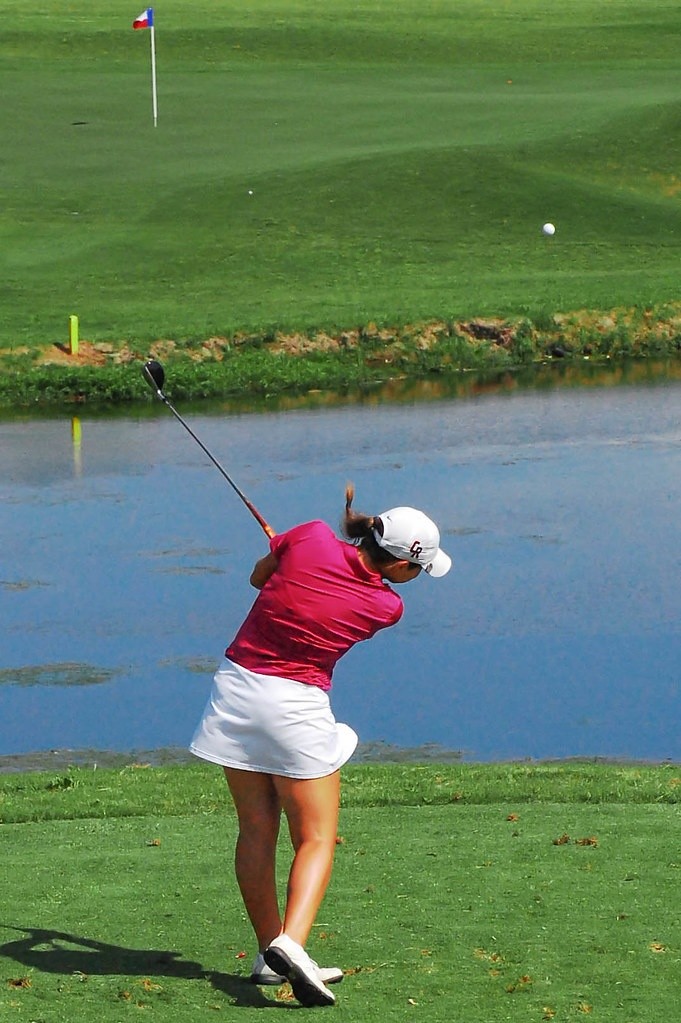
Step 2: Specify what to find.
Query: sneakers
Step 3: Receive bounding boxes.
[250,934,343,1005]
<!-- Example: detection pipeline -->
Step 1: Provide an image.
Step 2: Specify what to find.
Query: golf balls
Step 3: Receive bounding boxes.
[543,223,555,237]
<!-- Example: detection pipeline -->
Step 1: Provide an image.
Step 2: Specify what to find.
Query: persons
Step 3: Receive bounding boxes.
[187,483,453,1009]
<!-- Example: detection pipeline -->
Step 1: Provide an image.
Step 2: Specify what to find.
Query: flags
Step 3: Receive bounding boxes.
[133,7,154,29]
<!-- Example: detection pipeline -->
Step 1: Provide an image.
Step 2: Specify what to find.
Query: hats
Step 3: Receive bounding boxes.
[370,506,452,578]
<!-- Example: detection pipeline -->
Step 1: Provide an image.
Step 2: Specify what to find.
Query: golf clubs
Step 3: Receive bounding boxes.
[142,360,278,539]
